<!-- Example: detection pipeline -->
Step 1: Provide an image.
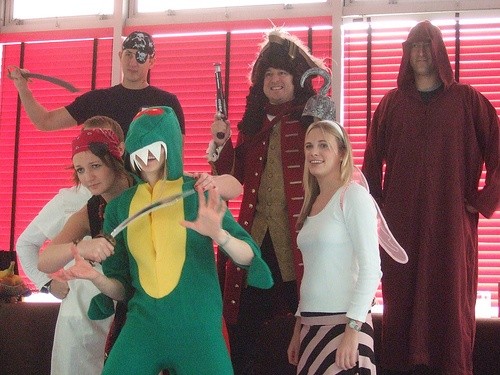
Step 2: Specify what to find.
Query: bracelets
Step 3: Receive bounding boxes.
[73,240,95,268]
[216,231,230,246]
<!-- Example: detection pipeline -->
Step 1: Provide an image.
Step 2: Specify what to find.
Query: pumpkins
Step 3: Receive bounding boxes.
[0,261,24,295]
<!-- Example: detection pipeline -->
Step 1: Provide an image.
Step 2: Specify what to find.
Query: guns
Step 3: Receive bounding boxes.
[212,62,228,139]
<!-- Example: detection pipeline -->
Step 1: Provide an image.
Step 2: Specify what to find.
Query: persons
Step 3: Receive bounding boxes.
[288,120,408,375]
[15,115,126,375]
[64,107,273,375]
[38,114,241,375]
[362,22,500,375]
[203,27,336,375]
[12,31,188,135]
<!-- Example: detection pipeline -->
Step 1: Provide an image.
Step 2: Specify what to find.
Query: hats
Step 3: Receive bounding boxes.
[72,129,122,161]
[250,29,329,85]
[122,31,154,56]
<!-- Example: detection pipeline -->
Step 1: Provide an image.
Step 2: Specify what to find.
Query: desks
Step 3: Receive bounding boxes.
[0,291,61,375]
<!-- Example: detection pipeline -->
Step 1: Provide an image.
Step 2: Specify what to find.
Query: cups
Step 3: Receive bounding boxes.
[475,290,492,319]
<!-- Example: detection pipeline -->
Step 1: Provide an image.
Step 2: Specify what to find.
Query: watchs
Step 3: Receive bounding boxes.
[346,319,361,332]
